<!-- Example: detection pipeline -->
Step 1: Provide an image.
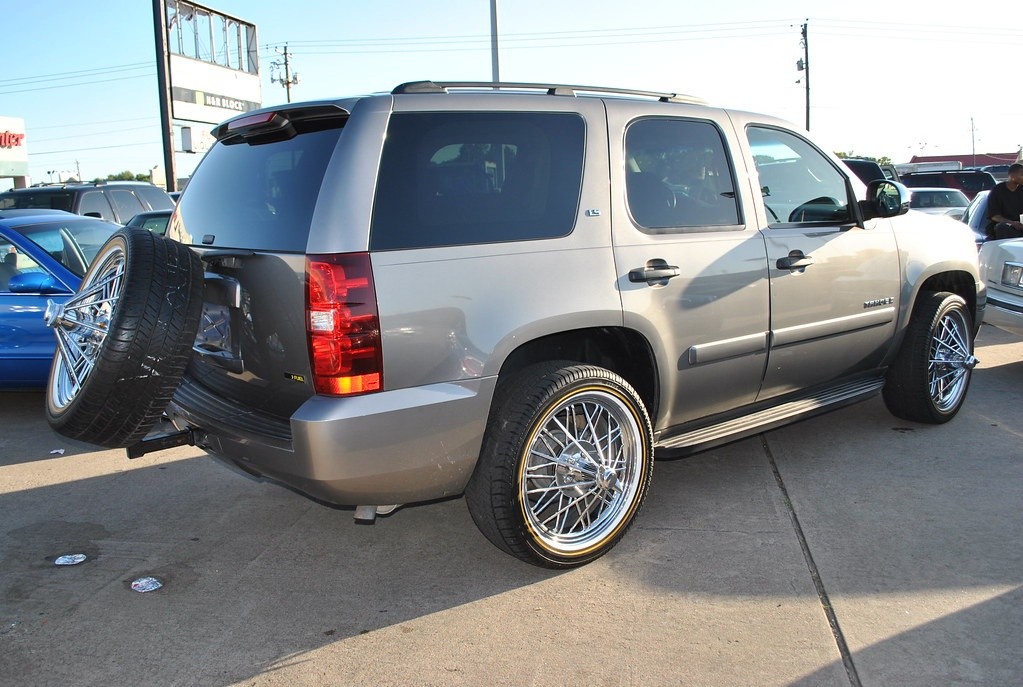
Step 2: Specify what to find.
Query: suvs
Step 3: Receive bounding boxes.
[0,177,184,235]
[43,79,994,569]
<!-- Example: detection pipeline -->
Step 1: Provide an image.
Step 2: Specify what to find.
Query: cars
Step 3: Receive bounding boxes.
[839,157,1023,343]
[0,208,126,390]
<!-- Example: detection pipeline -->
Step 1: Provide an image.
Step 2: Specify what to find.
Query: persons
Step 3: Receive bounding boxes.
[984,163,1023,241]
